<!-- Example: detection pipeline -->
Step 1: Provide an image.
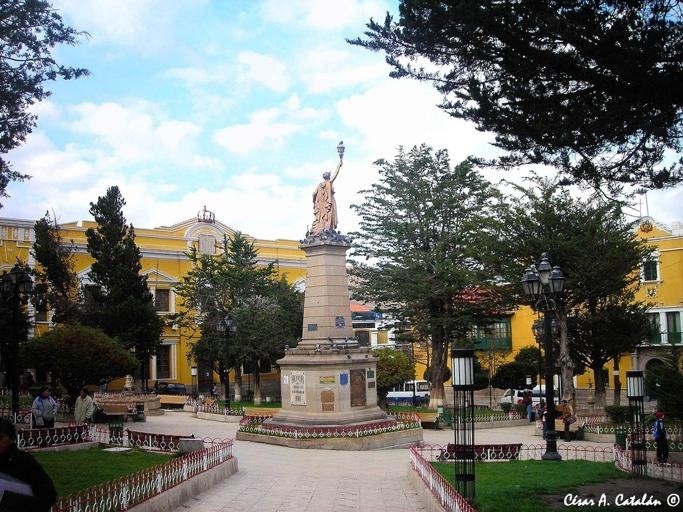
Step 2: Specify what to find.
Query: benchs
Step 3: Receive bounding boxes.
[399,410,441,431]
[237,405,277,426]
[436,441,523,464]
[123,427,196,452]
[156,392,189,407]
[96,402,137,423]
[16,422,89,450]
[198,396,215,408]
[538,417,588,441]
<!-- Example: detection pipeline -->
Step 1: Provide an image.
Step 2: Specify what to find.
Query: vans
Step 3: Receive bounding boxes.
[500,382,558,415]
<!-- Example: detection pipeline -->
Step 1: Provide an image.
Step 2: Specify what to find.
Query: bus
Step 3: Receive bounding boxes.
[382,379,431,408]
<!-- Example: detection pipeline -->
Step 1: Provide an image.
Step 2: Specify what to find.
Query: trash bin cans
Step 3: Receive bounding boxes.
[109,421,123,446]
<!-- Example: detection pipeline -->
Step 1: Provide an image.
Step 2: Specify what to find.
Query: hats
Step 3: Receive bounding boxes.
[656,412,664,418]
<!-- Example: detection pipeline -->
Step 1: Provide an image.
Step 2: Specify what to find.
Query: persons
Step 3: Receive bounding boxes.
[652,411,671,465]
[210,382,218,399]
[0,416,57,511]
[312,159,343,234]
[30,387,57,426]
[521,390,572,441]
[72,388,94,427]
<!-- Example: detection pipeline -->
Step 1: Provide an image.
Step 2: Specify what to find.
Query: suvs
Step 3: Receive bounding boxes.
[143,381,189,396]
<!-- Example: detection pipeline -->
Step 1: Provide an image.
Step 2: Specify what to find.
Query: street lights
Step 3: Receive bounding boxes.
[525,375,532,392]
[189,366,198,396]
[448,332,477,505]
[217,315,239,415]
[0,264,34,415]
[624,370,646,477]
[521,250,568,462]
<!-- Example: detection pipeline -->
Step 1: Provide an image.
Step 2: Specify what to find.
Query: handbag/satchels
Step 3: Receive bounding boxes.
[566,416,577,425]
